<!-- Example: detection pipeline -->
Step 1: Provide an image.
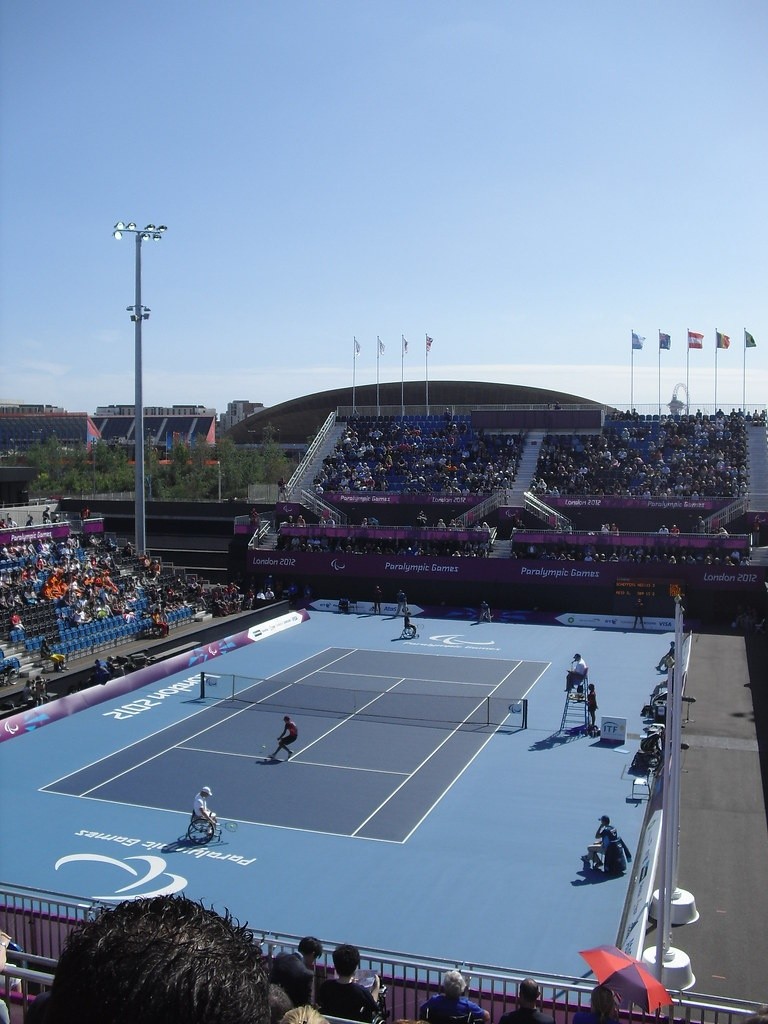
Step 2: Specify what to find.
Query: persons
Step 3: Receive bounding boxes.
[373,586,417,636]
[479,601,488,620]
[732,602,758,631]
[555,401,562,409]
[287,581,348,613]
[565,653,588,694]
[274,406,516,557]
[745,410,766,426]
[580,598,676,870]
[268,716,298,759]
[612,407,640,421]
[695,408,744,419]
[0,505,276,707]
[0,891,620,1024]
[516,415,768,565]
[195,787,217,836]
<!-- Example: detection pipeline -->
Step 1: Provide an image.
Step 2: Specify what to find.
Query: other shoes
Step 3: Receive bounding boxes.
[268,755,275,759]
[582,855,590,862]
[288,751,293,758]
[595,861,604,868]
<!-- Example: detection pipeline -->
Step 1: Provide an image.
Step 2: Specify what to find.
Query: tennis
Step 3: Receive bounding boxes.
[230,825,234,828]
[262,746,266,748]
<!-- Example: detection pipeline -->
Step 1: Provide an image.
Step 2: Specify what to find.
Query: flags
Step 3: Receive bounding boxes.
[717,332,730,349]
[745,331,756,347]
[403,338,408,354]
[659,333,671,350]
[380,340,385,356]
[632,332,646,350]
[355,340,360,358]
[426,336,434,351]
[688,332,704,349]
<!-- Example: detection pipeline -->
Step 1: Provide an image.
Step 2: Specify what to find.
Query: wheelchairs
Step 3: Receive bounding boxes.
[148,621,168,639]
[401,626,419,639]
[317,976,390,1024]
[0,668,19,687]
[419,1007,483,1024]
[188,809,222,845]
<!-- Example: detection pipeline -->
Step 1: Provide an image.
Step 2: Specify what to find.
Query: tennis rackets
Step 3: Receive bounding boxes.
[215,821,238,832]
[416,624,424,629]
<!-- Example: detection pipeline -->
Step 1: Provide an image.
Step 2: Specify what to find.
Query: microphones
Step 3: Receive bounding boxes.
[572,660,576,663]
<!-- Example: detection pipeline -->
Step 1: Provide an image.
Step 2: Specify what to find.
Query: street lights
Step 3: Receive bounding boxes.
[112,222,167,551]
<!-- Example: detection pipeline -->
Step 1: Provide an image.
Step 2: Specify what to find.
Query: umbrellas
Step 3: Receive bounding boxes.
[577,945,676,1014]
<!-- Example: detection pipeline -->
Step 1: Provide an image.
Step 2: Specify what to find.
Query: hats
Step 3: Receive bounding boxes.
[202,787,212,796]
[598,816,609,821]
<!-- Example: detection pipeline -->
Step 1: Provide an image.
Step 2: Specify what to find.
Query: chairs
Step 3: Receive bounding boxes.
[632,769,652,800]
[0,534,194,676]
[276,414,767,571]
[593,837,632,873]
